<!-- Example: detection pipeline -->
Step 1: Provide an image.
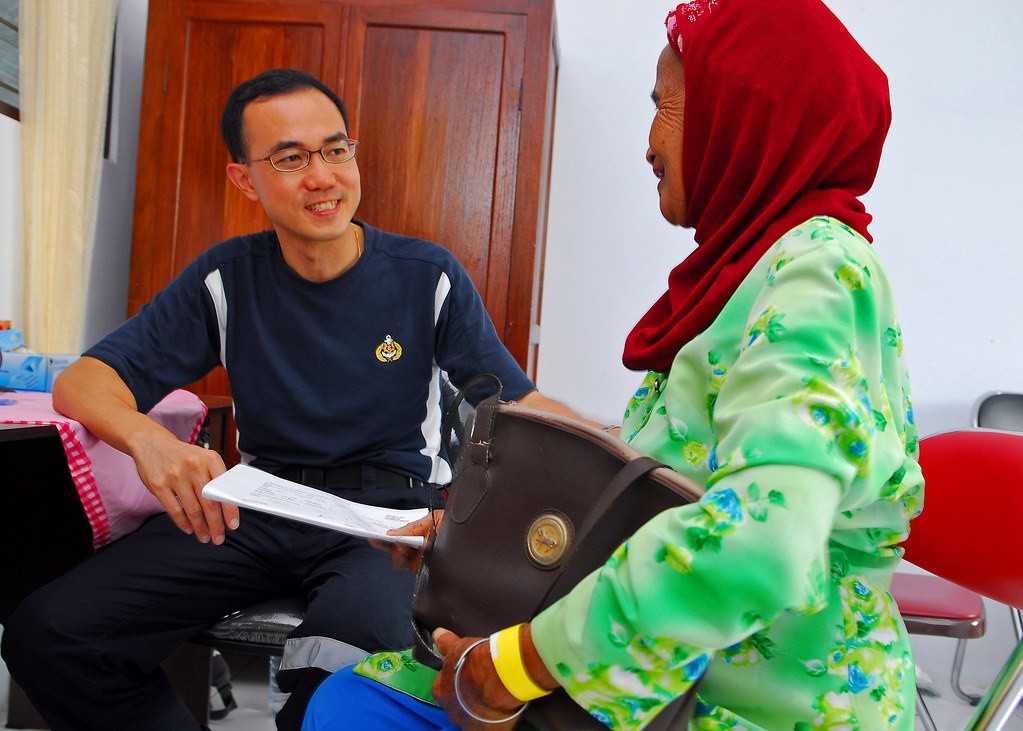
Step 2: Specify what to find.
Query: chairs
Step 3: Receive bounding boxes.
[894,426,1023,731]
[889,572,986,731]
[185,367,461,661]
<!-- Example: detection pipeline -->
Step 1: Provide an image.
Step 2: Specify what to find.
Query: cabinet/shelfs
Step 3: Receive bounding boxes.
[126,0,560,470]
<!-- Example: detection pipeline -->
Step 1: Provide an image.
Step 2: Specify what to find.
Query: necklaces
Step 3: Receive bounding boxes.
[351,223,361,259]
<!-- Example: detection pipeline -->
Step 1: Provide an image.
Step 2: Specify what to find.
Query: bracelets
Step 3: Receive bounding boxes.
[454,638,529,723]
[489,623,555,702]
[603,425,622,433]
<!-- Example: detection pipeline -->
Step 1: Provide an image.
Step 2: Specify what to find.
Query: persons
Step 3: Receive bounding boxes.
[0,67,621,731]
[302,0,924,731]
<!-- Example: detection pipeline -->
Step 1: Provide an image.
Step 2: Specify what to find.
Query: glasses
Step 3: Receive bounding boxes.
[239,139,358,172]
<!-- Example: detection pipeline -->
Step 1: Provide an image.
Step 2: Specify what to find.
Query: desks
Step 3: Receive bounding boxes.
[0,391,232,628]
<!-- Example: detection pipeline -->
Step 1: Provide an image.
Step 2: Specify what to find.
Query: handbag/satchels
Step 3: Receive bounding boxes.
[410,373,704,731]
